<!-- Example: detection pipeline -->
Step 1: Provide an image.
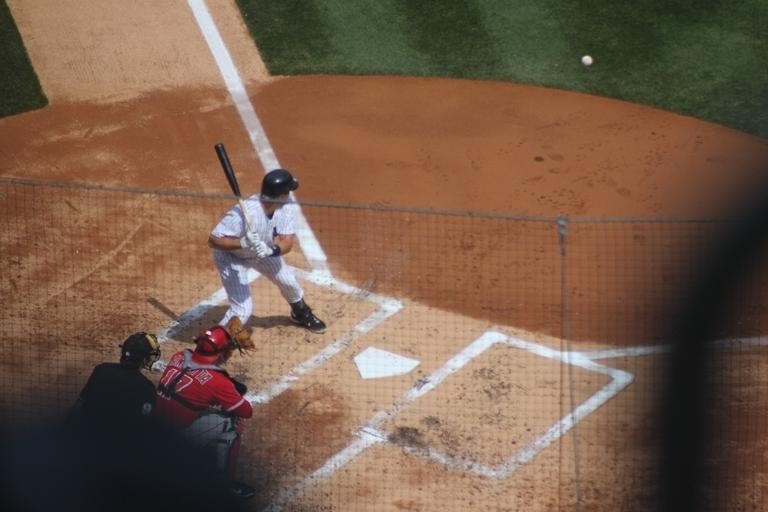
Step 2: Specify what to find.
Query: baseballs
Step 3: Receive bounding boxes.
[580,55,592,65]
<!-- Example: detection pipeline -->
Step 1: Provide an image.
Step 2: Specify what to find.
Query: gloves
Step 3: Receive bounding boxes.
[241,229,259,250]
[251,241,275,260]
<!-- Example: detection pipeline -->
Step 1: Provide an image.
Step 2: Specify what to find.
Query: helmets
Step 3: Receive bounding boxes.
[260,170,300,203]
[193,326,235,367]
[119,330,161,373]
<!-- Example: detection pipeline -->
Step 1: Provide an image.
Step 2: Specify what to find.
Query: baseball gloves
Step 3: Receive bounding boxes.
[224,316,255,351]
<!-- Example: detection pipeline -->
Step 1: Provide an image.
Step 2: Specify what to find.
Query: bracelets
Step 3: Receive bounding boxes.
[270,243,281,257]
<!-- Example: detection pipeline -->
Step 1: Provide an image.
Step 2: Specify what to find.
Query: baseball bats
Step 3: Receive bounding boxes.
[213,142,255,231]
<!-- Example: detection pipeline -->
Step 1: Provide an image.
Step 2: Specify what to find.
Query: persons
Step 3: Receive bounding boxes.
[152,314,257,500]
[51,327,163,493]
[207,169,328,334]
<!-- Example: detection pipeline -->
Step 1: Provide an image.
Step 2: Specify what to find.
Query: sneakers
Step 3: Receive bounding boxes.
[290,307,328,334]
[228,482,255,500]
